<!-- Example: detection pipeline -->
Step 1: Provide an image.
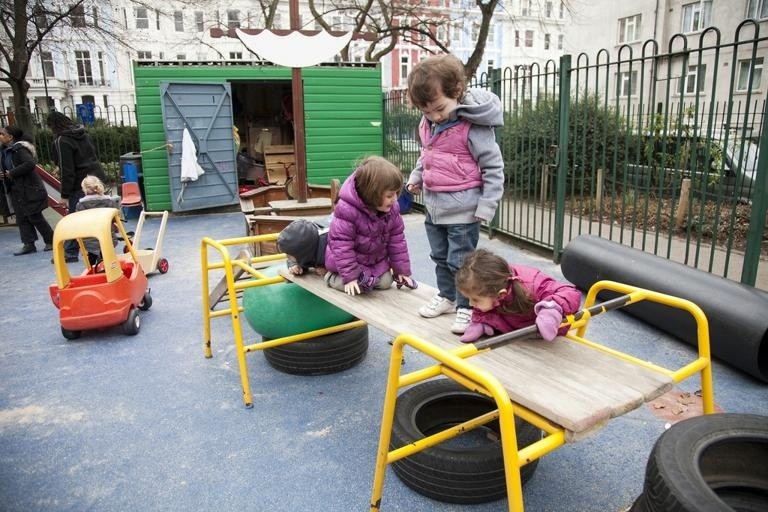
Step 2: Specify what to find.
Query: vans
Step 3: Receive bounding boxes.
[607,124,760,207]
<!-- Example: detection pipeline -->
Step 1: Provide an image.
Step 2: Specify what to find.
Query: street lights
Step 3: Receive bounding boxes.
[32,0,48,113]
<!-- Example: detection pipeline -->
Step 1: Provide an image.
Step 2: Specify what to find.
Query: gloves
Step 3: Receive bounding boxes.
[534,299,563,342]
[391,272,418,289]
[358,273,380,295]
[459,322,494,343]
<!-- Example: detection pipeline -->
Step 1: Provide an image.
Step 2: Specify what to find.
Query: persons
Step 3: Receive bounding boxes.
[404,55,505,336]
[323,155,414,296]
[75,174,119,268]
[0,125,54,257]
[455,248,584,342]
[274,213,334,277]
[46,111,109,264]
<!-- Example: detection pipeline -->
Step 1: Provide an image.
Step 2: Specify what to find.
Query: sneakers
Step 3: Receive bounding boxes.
[50,256,79,264]
[451,307,473,333]
[418,291,458,317]
[324,271,334,288]
[14,242,37,256]
[44,244,52,251]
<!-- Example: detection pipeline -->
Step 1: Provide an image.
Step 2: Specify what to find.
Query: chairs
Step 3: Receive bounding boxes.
[120,182,145,222]
